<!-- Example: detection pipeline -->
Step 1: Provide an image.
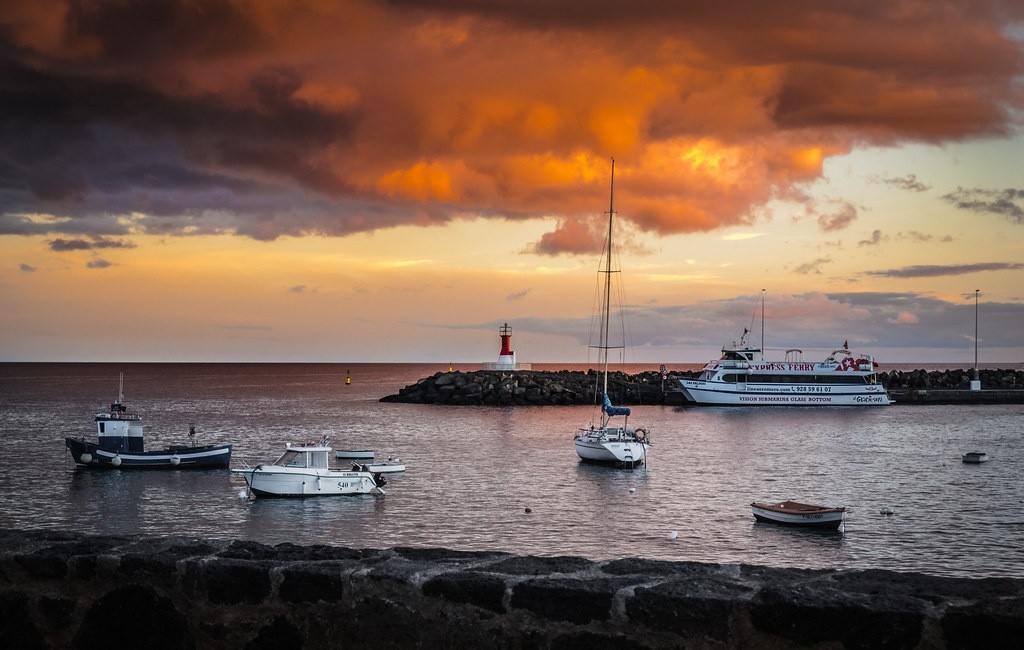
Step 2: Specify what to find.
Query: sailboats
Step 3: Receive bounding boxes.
[574,158,651,469]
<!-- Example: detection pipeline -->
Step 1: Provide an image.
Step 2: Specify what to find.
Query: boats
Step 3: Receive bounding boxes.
[360,457,405,473]
[750,500,846,531]
[65,371,233,471]
[232,435,387,499]
[671,289,896,406]
[962,452,989,464]
[335,449,374,460]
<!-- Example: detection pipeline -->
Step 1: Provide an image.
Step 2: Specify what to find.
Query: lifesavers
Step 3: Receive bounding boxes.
[634,428,646,442]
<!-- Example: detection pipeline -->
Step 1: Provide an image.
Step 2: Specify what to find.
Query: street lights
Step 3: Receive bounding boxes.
[969,290,981,381]
